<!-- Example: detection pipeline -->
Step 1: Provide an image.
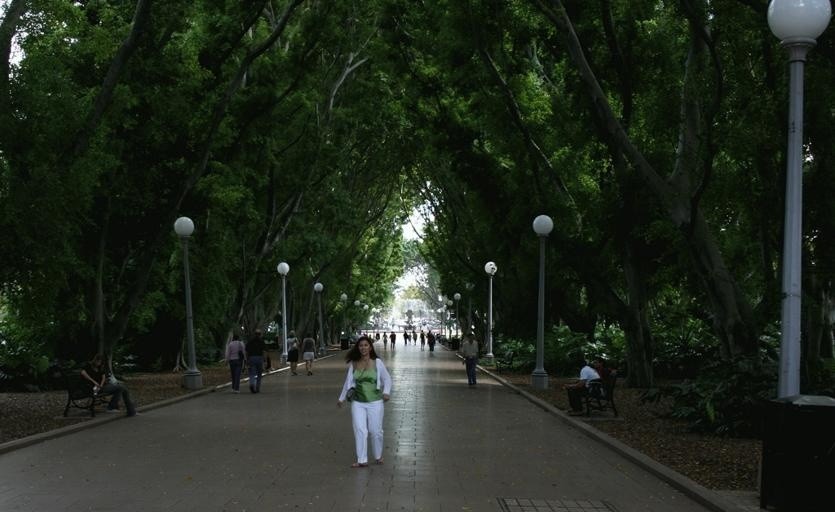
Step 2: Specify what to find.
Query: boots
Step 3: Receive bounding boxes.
[376,457,384,465]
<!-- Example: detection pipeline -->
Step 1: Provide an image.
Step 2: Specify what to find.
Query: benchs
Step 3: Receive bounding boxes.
[63,372,115,418]
[497,349,515,373]
[579,368,618,415]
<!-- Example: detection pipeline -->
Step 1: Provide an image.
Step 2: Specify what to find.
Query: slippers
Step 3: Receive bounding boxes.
[351,462,367,467]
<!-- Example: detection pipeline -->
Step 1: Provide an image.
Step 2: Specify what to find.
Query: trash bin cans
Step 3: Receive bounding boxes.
[451,338,459,350]
[760,395,835,512]
[340,338,348,350]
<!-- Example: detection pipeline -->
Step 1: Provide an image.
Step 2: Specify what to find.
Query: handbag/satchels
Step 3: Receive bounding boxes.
[346,388,355,401]
[293,343,298,349]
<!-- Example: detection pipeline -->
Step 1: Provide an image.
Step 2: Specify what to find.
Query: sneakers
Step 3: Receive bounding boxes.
[568,408,584,416]
[232,390,240,394]
[291,372,297,375]
[307,371,312,376]
[106,409,120,414]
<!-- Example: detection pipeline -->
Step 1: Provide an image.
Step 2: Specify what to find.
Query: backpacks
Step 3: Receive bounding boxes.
[239,350,244,359]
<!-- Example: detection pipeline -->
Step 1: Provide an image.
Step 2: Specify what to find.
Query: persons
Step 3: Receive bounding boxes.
[80,353,142,416]
[560,360,609,416]
[224,329,316,393]
[463,334,479,385]
[383,329,436,351]
[337,336,392,467]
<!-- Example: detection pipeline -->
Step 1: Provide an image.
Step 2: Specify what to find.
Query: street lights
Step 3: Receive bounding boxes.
[439,299,453,348]
[764,2,831,398]
[354,300,380,344]
[314,283,327,354]
[341,293,349,349]
[484,260,497,368]
[454,291,462,349]
[277,262,290,366]
[174,217,204,392]
[530,214,555,390]
